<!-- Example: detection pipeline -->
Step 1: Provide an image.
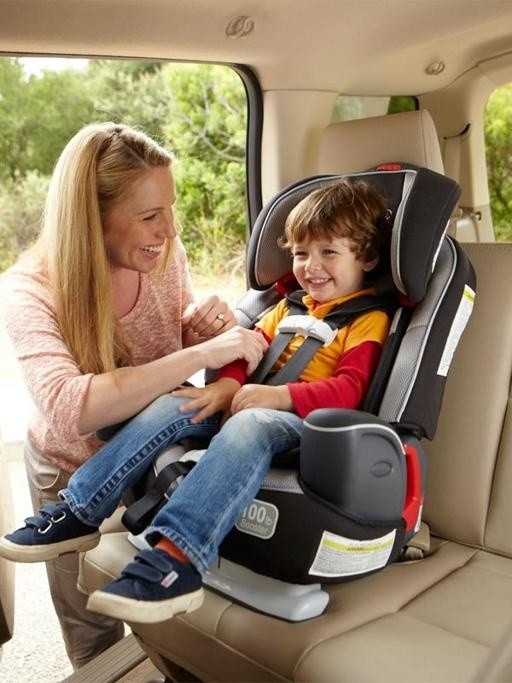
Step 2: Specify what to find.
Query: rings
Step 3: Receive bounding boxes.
[217,314,225,326]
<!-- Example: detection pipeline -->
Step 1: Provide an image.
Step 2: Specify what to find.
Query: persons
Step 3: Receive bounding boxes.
[1,121,270,673]
[0,175,393,624]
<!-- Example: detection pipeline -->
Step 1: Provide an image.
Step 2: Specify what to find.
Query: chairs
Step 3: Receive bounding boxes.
[76,109,509,682]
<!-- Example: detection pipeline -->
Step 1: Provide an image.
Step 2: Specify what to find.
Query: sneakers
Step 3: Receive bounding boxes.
[0,501,100,561]
[85,547,205,623]
